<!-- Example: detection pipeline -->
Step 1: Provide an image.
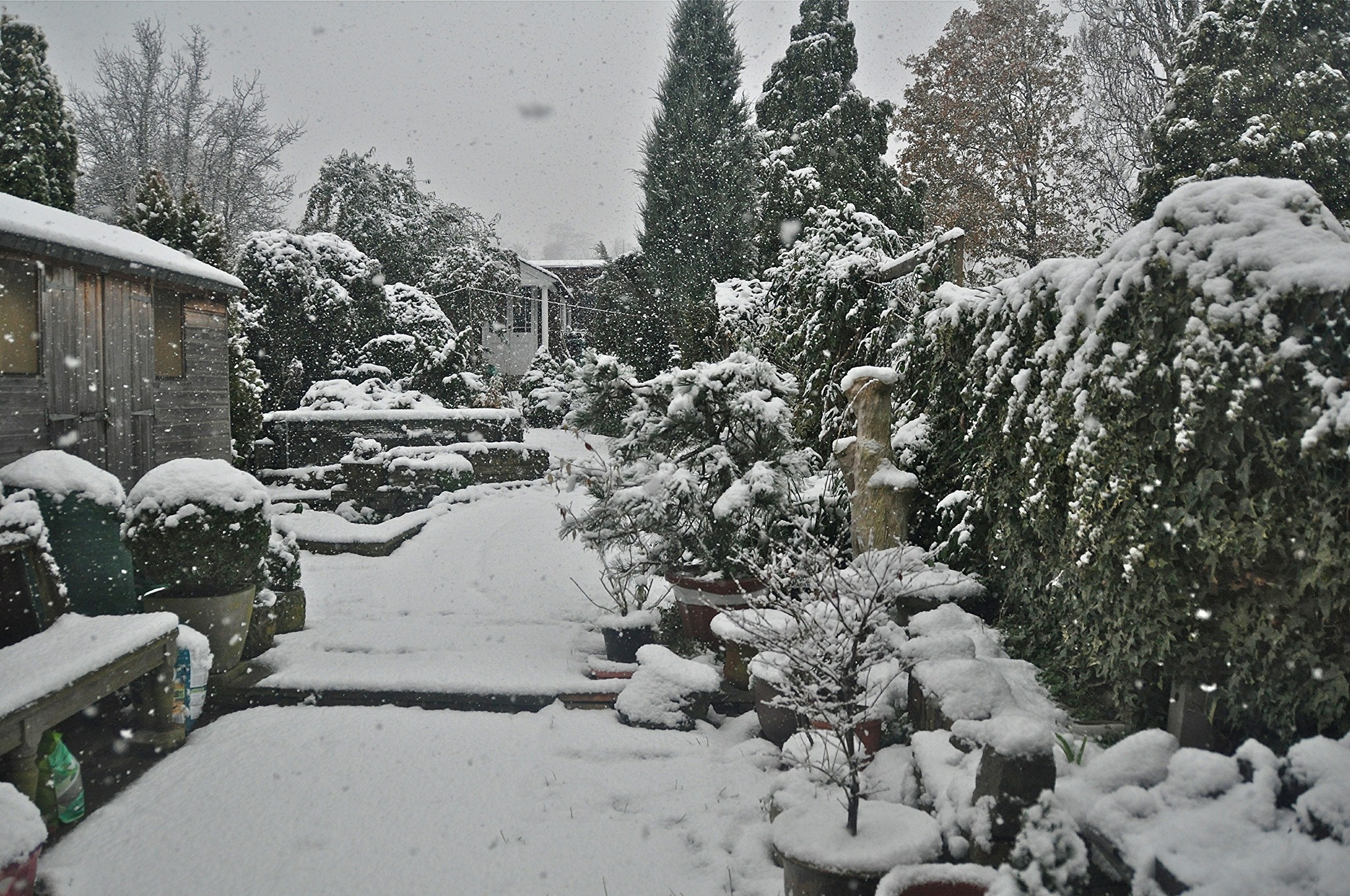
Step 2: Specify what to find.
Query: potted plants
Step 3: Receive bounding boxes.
[0,445,308,688]
[554,343,990,894]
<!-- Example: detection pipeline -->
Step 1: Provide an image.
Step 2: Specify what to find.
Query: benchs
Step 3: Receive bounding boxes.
[0,487,182,799]
